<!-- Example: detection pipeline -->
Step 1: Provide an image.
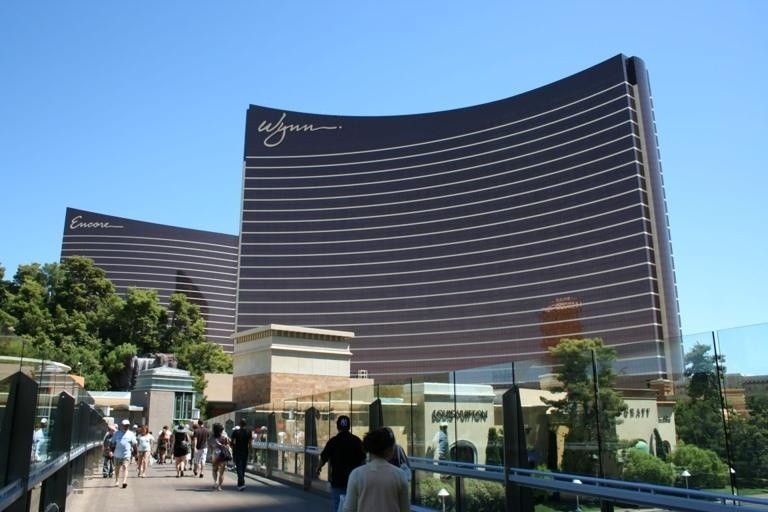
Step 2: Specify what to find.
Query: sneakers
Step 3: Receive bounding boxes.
[214,480,222,490]
[176,468,203,477]
[175,423,189,432]
[237,486,246,490]
[232,426,240,430]
[136,467,145,477]
[115,481,128,488]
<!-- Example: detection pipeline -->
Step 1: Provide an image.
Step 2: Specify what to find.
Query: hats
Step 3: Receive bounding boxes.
[337,415,350,431]
[440,422,448,426]
[122,420,130,425]
[132,425,137,429]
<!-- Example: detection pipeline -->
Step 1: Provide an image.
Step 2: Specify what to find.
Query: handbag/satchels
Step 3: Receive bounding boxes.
[154,451,158,460]
[181,441,190,450]
[400,462,411,480]
[223,445,232,460]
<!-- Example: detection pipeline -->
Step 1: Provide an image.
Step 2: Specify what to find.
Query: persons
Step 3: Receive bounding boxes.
[208,423,232,491]
[230,418,253,491]
[250,420,306,477]
[379,432,410,475]
[314,413,368,512]
[33,417,49,463]
[432,421,452,480]
[100,417,212,489]
[340,427,411,511]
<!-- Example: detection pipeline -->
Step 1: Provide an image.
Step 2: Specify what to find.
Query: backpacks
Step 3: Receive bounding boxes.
[159,432,168,442]
